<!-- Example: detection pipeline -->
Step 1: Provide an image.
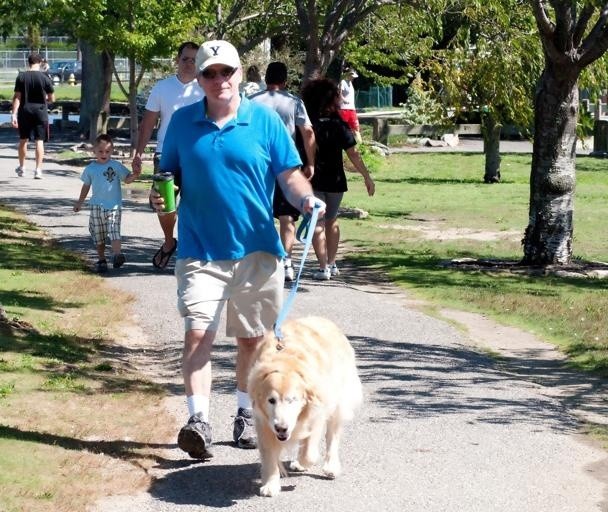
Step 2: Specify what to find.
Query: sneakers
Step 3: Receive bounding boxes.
[284,262,295,283]
[14,166,24,177]
[33,168,42,180]
[112,253,126,270]
[177,414,215,461]
[312,263,340,280]
[231,407,257,450]
[93,259,109,273]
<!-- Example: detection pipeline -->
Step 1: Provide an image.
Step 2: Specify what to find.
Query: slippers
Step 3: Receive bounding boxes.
[152,238,177,269]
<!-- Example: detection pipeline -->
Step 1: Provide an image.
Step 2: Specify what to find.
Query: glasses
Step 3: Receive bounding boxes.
[202,66,236,79]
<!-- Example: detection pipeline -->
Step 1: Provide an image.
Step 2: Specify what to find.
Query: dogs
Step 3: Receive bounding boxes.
[248,315,363,498]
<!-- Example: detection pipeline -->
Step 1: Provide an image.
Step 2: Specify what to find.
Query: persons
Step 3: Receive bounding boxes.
[10,52,57,180]
[41,58,53,80]
[132,41,207,269]
[239,64,268,98]
[73,133,141,273]
[336,66,362,147]
[300,78,376,279]
[248,61,317,281]
[151,39,327,459]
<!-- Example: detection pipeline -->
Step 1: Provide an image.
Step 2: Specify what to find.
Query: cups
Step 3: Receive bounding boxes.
[153,171,176,213]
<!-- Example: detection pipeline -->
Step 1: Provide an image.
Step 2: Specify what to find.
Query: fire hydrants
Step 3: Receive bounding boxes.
[67,73,76,87]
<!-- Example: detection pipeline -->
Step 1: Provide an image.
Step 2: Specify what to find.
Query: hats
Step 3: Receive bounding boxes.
[192,38,242,72]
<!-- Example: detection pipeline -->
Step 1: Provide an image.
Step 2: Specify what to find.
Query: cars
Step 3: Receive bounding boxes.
[45,59,82,87]
[0,97,157,135]
